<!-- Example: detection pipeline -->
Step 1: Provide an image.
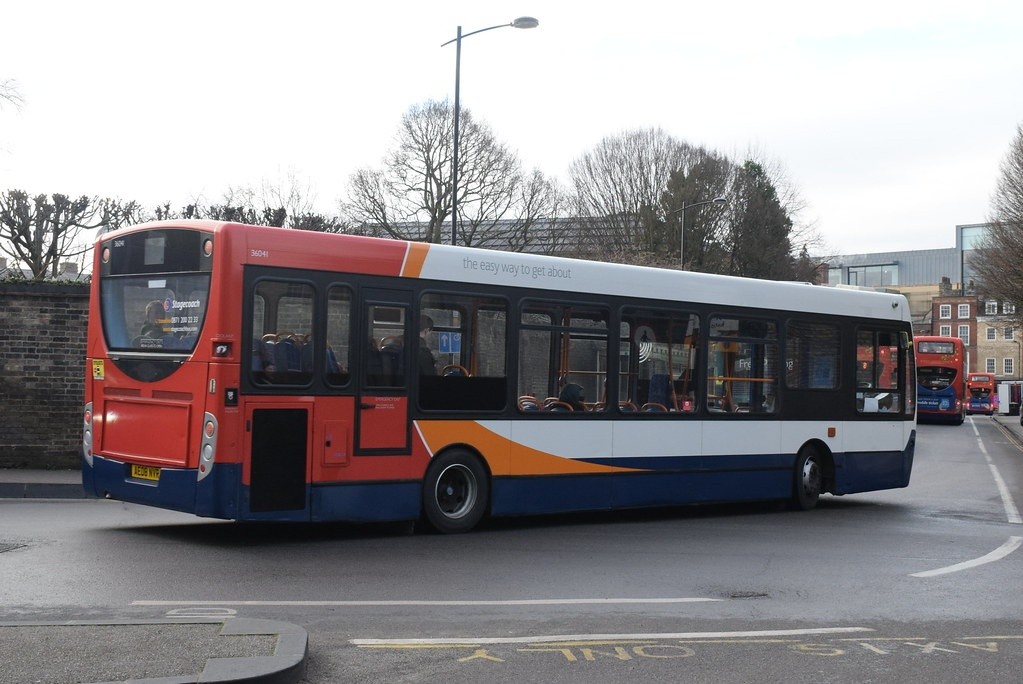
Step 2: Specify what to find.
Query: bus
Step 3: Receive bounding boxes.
[78,216,919,534]
[965,373,996,415]
[911,336,967,425]
[854,343,898,412]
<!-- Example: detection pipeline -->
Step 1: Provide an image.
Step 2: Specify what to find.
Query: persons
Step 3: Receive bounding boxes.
[519,380,643,411]
[251,333,346,374]
[368,314,436,390]
[141,301,165,338]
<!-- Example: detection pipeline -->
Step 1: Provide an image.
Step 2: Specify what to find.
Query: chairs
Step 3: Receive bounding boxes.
[137,332,339,376]
[376,336,473,377]
[517,395,667,411]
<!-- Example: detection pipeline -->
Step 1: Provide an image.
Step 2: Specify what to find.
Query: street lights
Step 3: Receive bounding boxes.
[672,198,728,269]
[440,16,540,243]
